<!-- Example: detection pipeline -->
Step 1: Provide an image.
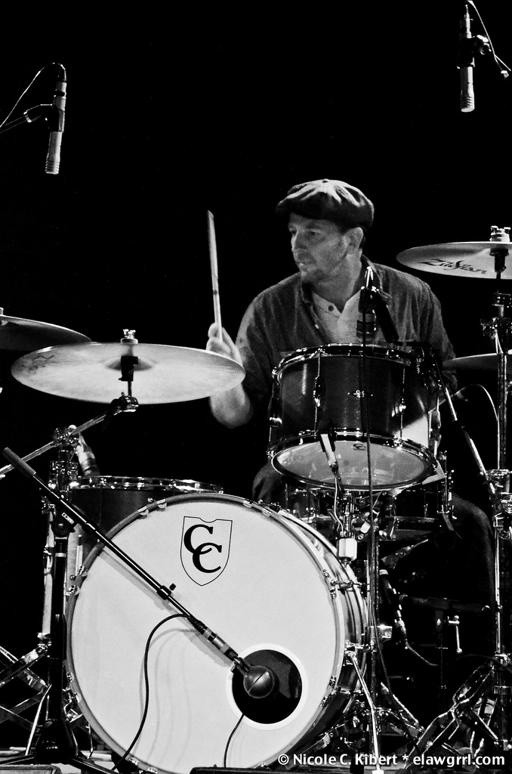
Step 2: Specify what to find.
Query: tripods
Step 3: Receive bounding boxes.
[0,249,512,774]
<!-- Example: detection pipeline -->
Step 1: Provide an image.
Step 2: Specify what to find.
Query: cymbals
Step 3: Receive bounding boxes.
[397,240,512,280]
[447,350,512,383]
[12,341,247,406]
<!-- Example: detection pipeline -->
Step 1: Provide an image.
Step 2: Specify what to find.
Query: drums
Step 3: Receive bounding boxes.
[56,477,222,540]
[67,493,367,772]
[264,345,440,496]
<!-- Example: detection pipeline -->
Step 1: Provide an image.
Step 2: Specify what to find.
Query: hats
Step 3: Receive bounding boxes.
[275,178,375,230]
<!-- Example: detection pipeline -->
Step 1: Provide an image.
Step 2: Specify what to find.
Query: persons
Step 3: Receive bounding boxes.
[206,177,496,668]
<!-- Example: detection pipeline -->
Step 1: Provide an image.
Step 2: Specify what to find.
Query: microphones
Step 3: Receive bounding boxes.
[45,64,67,175]
[459,4,476,112]
